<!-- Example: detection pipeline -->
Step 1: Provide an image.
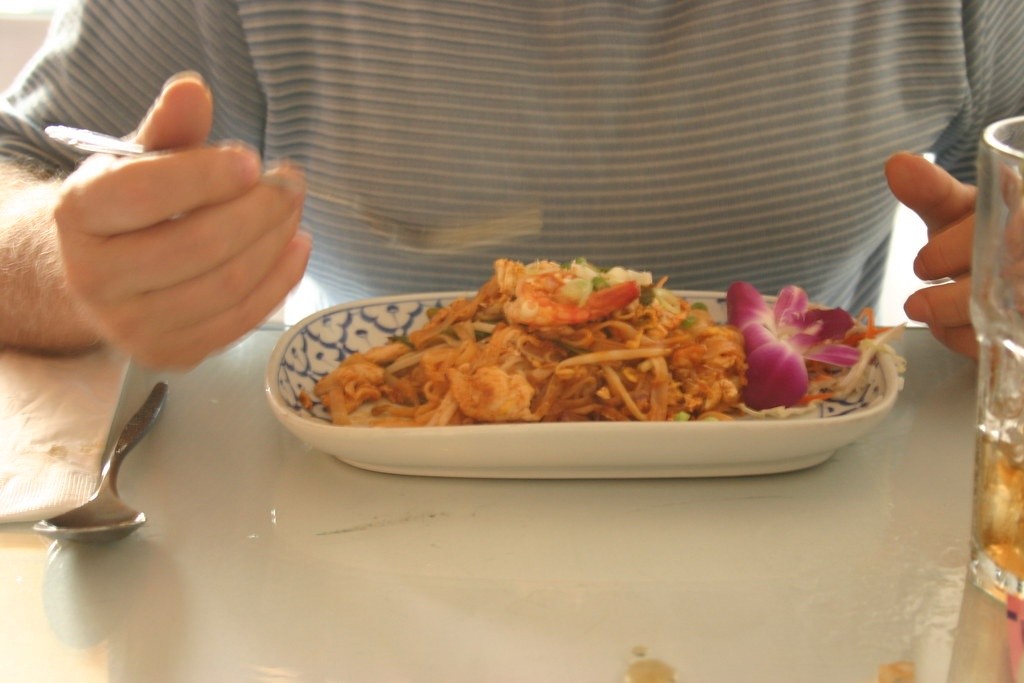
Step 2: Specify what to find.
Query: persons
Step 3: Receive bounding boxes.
[0,0,1024,372]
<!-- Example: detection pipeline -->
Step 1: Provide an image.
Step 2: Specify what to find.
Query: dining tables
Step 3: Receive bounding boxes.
[0,321,1011,683]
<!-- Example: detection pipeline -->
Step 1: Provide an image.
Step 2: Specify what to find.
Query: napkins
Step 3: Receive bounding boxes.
[0,343,133,525]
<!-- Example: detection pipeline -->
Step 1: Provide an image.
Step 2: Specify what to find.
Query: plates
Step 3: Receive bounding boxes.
[267,292,898,478]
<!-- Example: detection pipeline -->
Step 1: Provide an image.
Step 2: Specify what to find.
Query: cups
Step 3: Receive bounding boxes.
[966,118,1024,606]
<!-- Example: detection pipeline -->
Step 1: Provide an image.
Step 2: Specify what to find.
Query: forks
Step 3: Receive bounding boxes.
[44,124,541,257]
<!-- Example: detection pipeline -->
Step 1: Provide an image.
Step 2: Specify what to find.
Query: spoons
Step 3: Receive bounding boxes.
[33,383,169,545]
